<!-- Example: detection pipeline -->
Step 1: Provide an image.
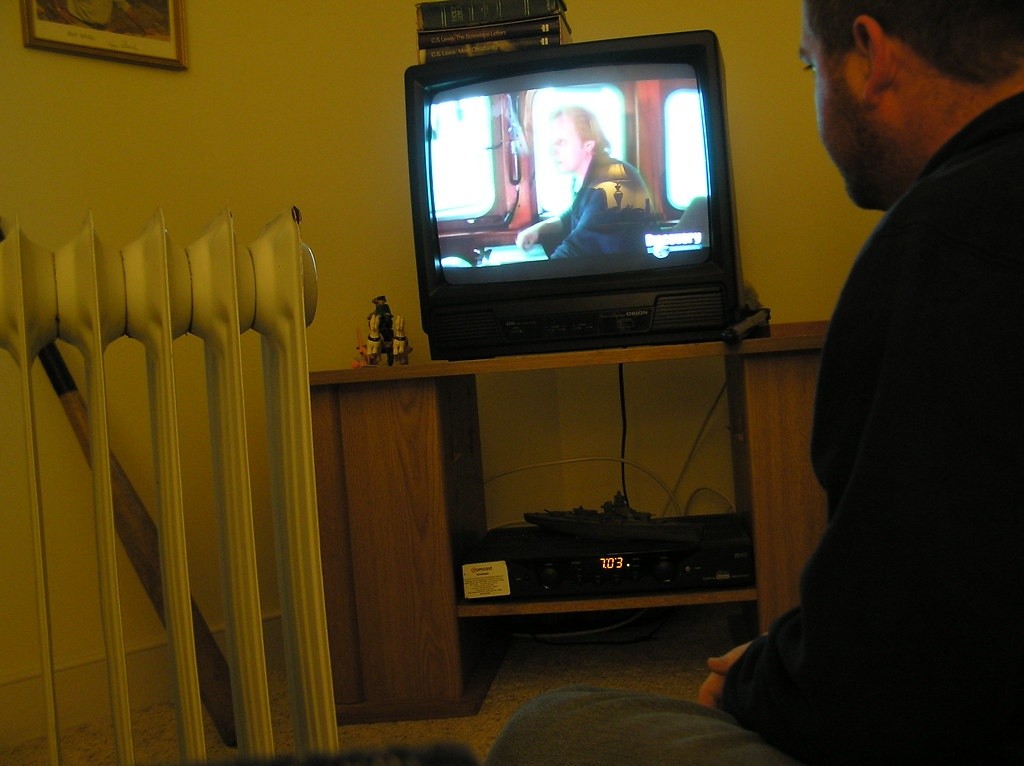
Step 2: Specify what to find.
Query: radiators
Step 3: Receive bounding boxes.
[1,206,340,765]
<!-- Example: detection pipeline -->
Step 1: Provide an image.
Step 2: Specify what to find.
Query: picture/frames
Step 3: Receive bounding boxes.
[19,0,190,71]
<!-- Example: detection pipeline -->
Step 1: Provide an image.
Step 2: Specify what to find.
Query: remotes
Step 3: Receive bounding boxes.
[524,511,705,543]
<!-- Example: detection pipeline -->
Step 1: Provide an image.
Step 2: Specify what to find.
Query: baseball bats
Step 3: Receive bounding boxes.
[0,225,242,750]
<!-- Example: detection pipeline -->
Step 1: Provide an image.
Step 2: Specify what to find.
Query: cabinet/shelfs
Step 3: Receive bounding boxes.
[307,319,831,726]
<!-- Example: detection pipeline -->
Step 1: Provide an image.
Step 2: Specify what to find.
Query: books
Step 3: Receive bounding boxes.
[417,1,574,64]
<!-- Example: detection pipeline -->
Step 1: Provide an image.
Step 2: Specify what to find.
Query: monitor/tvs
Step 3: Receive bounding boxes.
[403,28,744,362]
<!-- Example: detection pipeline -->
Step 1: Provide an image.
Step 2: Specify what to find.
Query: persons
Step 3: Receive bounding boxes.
[487,0,1023,764]
[514,107,664,263]
